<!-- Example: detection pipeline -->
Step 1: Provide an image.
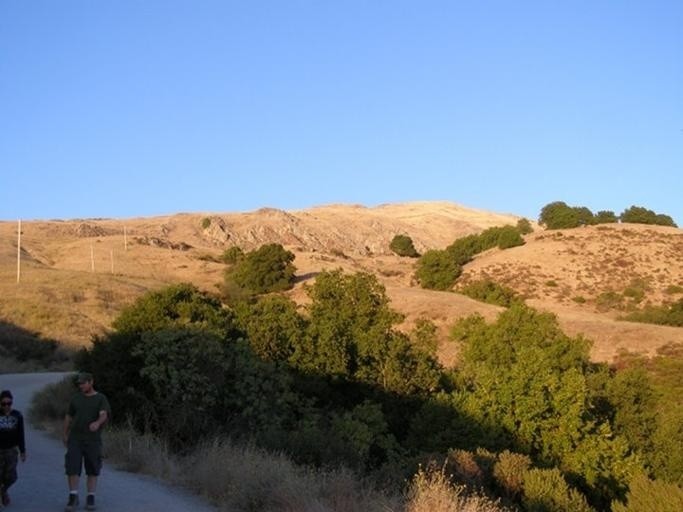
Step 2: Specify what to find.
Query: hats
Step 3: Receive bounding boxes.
[76,372,93,383]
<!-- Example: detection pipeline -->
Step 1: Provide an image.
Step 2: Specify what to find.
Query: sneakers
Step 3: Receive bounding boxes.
[66,493,80,511]
[86,494,96,510]
[3,495,10,505]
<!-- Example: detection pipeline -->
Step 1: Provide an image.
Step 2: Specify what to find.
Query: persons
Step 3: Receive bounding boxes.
[62,373,111,511]
[1,391,26,508]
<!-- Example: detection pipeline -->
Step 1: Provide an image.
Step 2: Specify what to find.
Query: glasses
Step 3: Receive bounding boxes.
[0,401,12,406]
